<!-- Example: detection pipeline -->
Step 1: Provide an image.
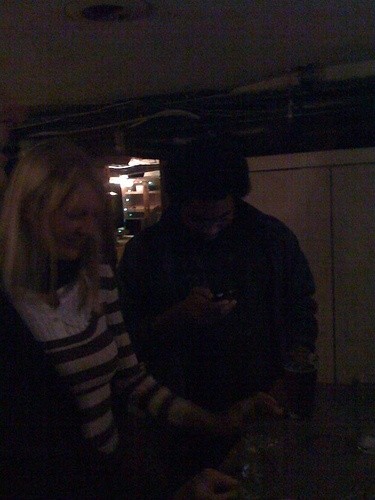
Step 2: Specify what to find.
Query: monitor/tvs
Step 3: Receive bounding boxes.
[125,219,140,235]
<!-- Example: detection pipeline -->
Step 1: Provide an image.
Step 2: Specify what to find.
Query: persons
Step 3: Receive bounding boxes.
[116,142,317,482]
[0,137,287,500]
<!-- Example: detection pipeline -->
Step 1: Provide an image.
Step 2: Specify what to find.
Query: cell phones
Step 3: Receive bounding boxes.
[215,288,235,301]
